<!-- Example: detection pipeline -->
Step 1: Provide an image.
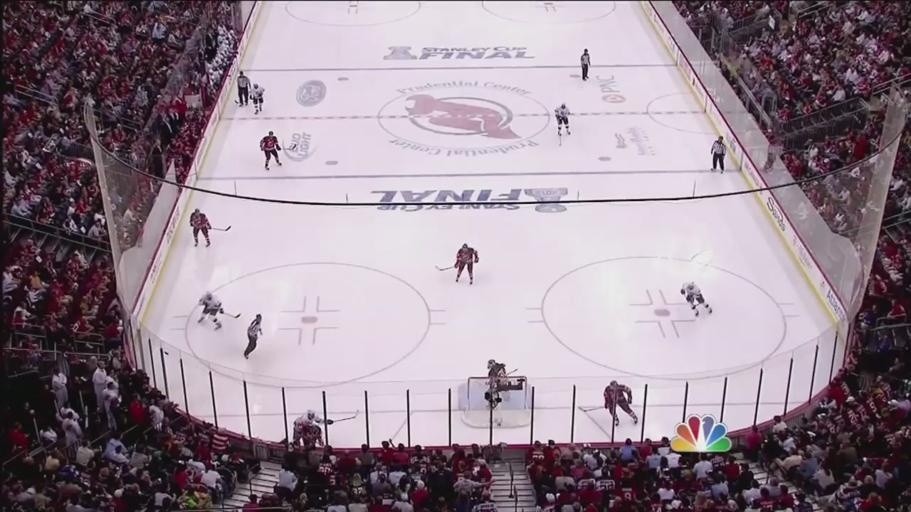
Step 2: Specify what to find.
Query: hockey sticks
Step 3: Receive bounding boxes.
[207,226,232,232]
[333,411,360,423]
[222,311,242,319]
[580,406,602,412]
[234,99,250,103]
[436,260,476,270]
[559,124,561,145]
[280,144,297,151]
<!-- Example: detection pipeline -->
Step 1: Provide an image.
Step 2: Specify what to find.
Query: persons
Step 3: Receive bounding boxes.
[581,48,592,82]
[260,130,283,171]
[248,83,265,115]
[556,103,572,136]
[670,0,911,511]
[604,380,638,427]
[0,0,250,511]
[244,312,263,360]
[484,358,511,407]
[454,243,481,285]
[250,407,670,512]
